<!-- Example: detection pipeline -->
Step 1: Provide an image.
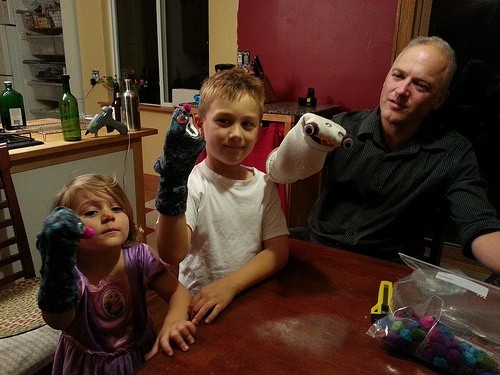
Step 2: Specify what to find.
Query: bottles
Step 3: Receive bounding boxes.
[57,75,81,142]
[304,87,317,107]
[0,80,26,130]
[111,83,121,122]
[119,79,142,132]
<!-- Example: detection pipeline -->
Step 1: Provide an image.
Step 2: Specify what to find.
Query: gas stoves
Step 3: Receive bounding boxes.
[0,128,44,153]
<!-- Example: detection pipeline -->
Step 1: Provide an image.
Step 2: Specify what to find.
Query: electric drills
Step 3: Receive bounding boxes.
[85,106,128,135]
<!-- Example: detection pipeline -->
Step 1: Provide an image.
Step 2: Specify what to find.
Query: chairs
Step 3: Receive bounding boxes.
[0,142,66,375]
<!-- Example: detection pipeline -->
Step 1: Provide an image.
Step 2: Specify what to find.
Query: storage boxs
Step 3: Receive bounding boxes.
[24,34,64,56]
[28,80,64,102]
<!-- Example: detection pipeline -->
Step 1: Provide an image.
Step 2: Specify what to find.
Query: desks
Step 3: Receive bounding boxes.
[260,99,345,229]
[131,237,500,375]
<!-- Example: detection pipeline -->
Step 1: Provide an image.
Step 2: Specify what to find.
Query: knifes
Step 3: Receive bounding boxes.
[248,55,264,79]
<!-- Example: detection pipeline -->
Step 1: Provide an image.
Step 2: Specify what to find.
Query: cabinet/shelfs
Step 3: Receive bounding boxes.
[20,26,69,104]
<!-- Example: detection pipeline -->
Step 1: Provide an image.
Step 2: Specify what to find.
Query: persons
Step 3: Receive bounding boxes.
[265,36,500,274]
[155,69,289,325]
[36,173,196,375]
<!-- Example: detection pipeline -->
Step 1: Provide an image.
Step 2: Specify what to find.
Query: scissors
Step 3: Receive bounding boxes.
[434,304,500,345]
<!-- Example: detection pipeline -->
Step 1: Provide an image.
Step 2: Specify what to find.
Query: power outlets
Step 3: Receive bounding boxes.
[91,70,99,83]
